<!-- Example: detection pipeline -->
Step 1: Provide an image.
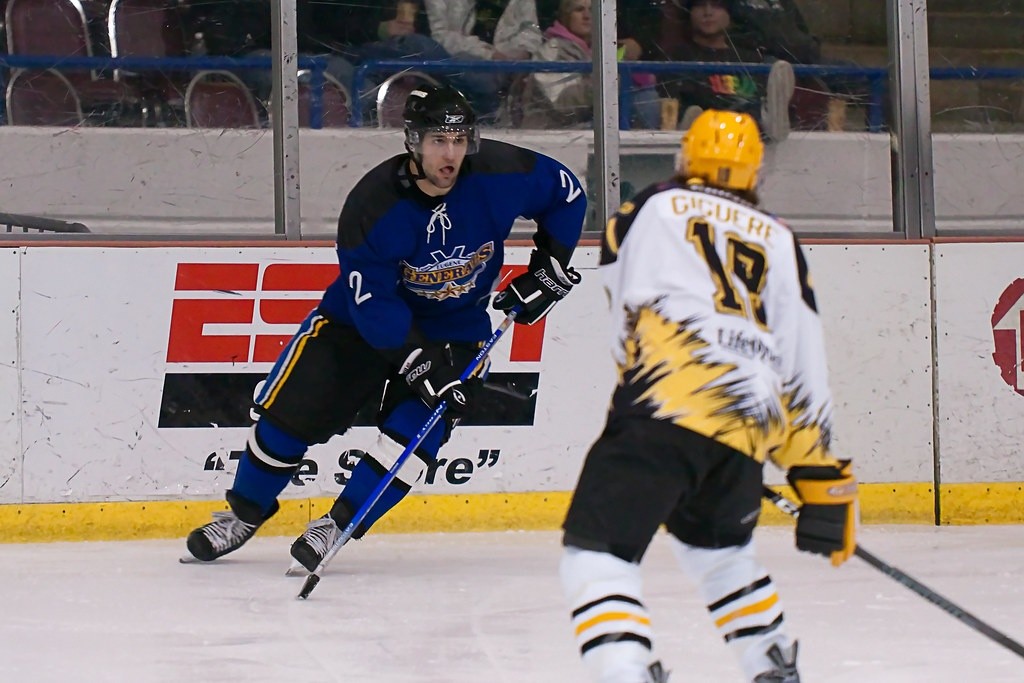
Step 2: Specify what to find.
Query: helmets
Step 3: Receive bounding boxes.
[675,110,765,192]
[403,83,477,153]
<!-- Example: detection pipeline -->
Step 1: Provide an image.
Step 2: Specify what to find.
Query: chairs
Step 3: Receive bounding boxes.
[0,0,847,133]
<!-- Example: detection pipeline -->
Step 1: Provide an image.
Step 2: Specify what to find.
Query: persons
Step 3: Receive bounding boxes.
[187,85,588,572]
[560,109,859,683]
[181,0,869,142]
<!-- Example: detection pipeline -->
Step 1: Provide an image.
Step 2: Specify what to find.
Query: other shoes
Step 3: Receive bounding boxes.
[760,61,796,141]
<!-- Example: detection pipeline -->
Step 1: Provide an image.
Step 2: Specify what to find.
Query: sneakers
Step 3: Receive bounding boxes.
[179,489,279,564]
[283,500,351,579]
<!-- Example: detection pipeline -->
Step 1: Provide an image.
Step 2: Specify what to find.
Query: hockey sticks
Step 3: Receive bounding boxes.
[761,481,1024,661]
[297,308,518,602]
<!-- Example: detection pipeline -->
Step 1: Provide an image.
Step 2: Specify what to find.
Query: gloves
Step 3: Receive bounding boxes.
[491,252,580,325]
[788,460,858,569]
[388,335,481,428]
